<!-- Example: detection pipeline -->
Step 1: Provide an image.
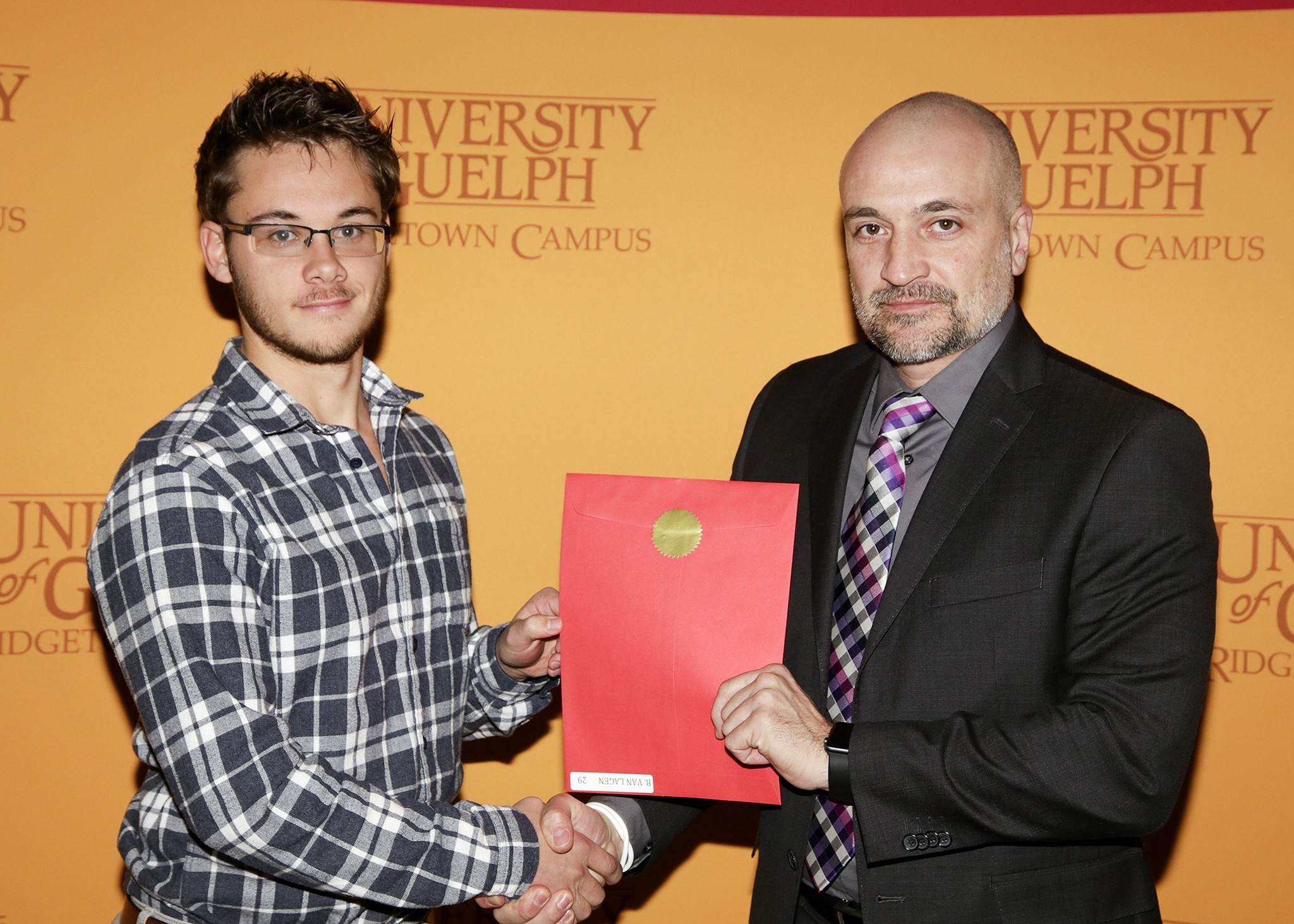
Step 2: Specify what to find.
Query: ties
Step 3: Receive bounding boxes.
[802,395,934,890]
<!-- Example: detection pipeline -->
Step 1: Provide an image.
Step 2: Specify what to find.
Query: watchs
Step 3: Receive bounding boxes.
[822,719,858,808]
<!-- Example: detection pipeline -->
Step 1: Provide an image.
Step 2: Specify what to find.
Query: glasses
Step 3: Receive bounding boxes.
[216,219,391,257]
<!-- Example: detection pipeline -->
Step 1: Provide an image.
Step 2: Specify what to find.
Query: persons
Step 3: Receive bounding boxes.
[85,66,626,924]
[469,90,1219,924]
